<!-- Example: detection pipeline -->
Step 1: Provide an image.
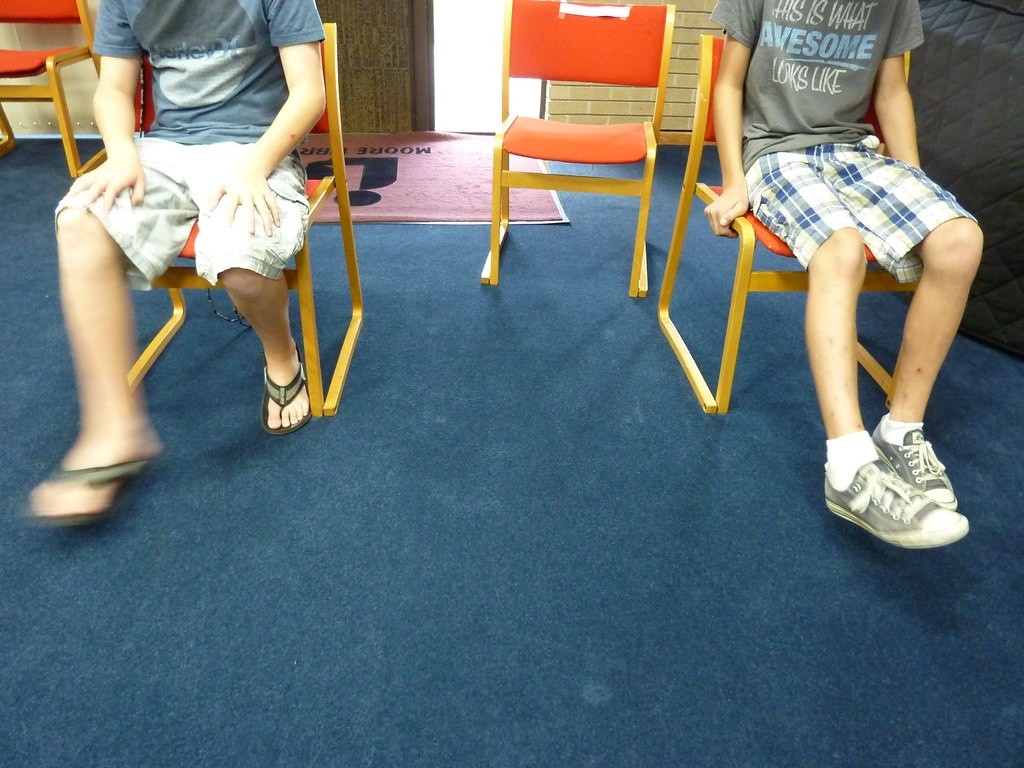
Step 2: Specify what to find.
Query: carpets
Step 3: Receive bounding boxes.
[299,132,570,224]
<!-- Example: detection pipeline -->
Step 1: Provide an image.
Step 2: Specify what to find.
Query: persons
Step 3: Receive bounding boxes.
[23,0,326,525]
[704,1,984,548]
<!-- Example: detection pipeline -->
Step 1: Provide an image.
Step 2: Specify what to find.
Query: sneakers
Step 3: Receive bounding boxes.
[823,456,969,549]
[871,413,958,515]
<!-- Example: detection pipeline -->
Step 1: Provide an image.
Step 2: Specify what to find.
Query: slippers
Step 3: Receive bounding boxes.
[258,342,313,435]
[17,452,152,529]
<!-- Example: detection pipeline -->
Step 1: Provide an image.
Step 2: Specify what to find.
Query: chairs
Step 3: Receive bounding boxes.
[657,35,922,416]
[0,0,107,178]
[480,0,679,297]
[127,21,364,418]
